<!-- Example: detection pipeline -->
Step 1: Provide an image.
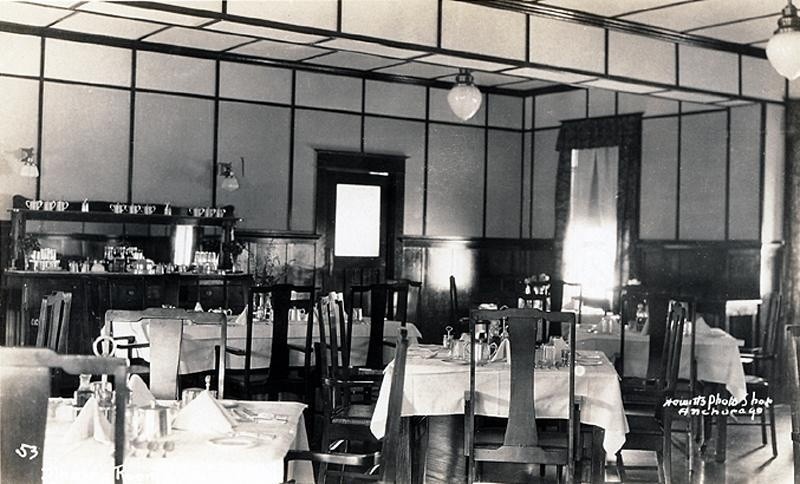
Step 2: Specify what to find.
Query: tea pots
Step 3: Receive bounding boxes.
[287,305,305,322]
[26,198,226,218]
[208,306,232,319]
[462,339,498,366]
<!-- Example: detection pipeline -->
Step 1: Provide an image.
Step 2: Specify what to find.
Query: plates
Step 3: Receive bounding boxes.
[208,435,258,449]
[441,358,465,365]
[576,358,604,366]
[216,399,239,409]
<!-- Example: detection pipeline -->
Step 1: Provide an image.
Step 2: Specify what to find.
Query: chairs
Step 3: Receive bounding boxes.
[0,272,800,484]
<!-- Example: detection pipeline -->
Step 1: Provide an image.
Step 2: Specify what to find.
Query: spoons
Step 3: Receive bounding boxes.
[232,407,287,424]
[130,441,174,458]
[404,351,439,358]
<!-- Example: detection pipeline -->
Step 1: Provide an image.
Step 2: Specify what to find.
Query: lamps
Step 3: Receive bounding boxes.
[765,0,800,81]
[218,162,239,192]
[19,147,39,185]
[447,67,482,121]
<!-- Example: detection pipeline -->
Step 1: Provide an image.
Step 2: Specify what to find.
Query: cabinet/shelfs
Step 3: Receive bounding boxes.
[7,194,243,272]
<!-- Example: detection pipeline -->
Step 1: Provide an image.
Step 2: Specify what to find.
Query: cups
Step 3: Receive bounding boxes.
[137,398,167,441]
